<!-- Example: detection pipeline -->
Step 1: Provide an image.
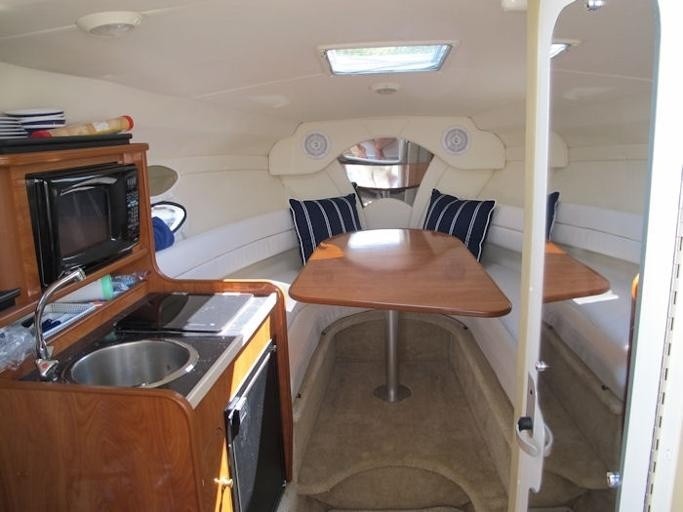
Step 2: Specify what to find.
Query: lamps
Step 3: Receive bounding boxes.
[77,10,143,38]
[317,40,456,80]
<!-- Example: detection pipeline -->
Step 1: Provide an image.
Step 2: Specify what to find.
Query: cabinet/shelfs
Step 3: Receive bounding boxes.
[193,376,234,512]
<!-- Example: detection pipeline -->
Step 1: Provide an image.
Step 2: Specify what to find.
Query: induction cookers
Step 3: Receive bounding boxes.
[112,292,254,334]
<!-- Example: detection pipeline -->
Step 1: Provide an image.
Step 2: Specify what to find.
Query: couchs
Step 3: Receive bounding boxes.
[543,205,648,420]
[156,198,523,407]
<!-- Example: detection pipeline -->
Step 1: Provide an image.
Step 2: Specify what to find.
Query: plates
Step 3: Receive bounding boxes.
[0,109,66,140]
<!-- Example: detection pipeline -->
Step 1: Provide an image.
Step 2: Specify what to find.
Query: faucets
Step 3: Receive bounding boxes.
[34,266,87,379]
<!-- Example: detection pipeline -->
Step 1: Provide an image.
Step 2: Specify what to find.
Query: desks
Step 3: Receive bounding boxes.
[542,241,609,303]
[288,229,511,402]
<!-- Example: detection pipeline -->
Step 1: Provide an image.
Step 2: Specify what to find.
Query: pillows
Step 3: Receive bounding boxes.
[546,191,560,241]
[288,192,362,267]
[422,188,495,263]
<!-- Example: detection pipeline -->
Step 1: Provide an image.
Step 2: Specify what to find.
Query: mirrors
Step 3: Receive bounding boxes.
[516,0,682,512]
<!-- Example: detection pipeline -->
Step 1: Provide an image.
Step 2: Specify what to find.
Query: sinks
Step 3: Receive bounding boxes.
[66,336,200,388]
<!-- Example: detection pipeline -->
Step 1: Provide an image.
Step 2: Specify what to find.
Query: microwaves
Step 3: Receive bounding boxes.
[25,161,140,292]
